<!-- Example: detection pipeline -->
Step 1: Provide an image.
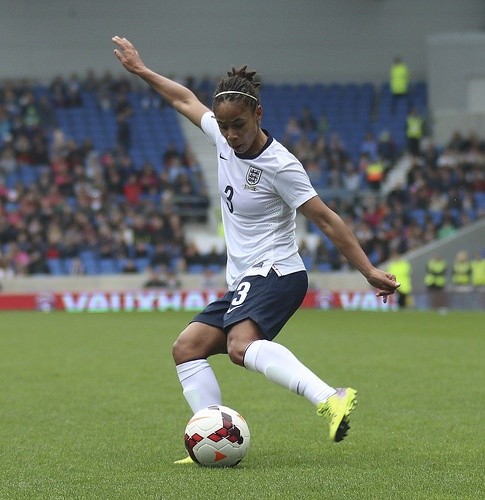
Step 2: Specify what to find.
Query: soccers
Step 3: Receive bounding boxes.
[184,404,250,467]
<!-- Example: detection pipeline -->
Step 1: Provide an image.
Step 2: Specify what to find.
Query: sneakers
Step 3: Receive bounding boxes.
[315,386,357,442]
[175,455,193,463]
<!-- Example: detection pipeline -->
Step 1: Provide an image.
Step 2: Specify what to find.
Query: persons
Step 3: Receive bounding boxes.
[111,34,401,465]
[0,67,485,311]
